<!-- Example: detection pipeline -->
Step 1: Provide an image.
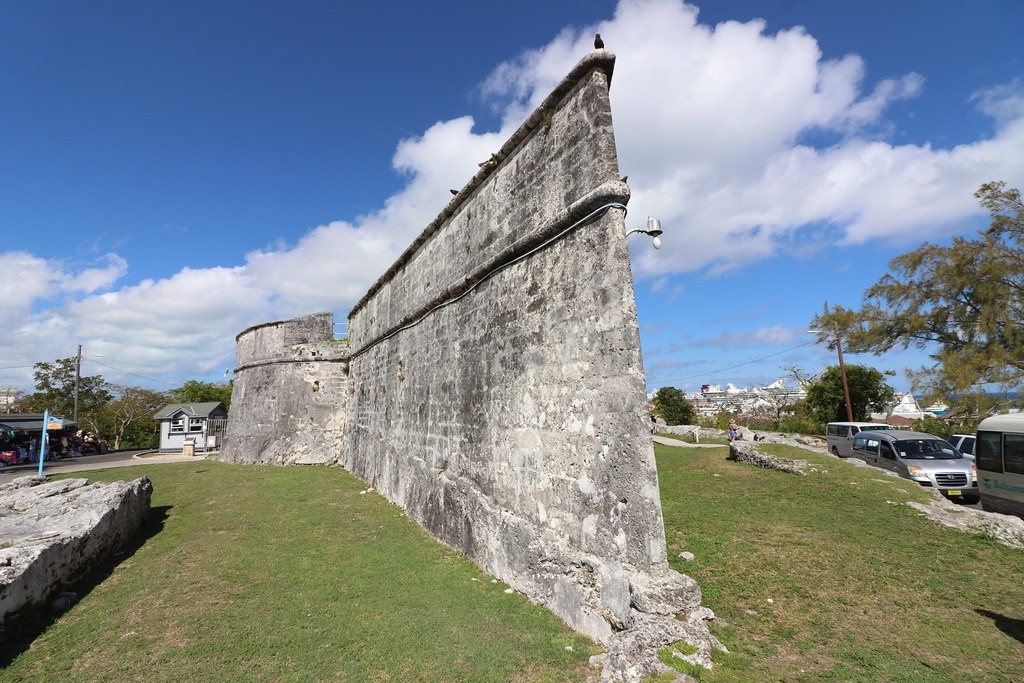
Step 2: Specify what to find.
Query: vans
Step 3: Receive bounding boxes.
[975,412,1023,521]
[850,430,980,505]
[949,433,981,504]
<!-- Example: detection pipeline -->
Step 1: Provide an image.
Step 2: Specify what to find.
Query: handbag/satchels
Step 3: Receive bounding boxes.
[733,425,736,430]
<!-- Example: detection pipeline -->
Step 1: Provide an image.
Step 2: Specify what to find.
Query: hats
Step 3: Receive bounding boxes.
[728,419,735,424]
[918,440,924,444]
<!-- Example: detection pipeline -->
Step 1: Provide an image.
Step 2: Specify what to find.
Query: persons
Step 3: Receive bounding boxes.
[728,419,738,442]
[738,432,765,442]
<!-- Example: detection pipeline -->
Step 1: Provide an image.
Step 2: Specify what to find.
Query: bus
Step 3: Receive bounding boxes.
[826,422,891,458]
